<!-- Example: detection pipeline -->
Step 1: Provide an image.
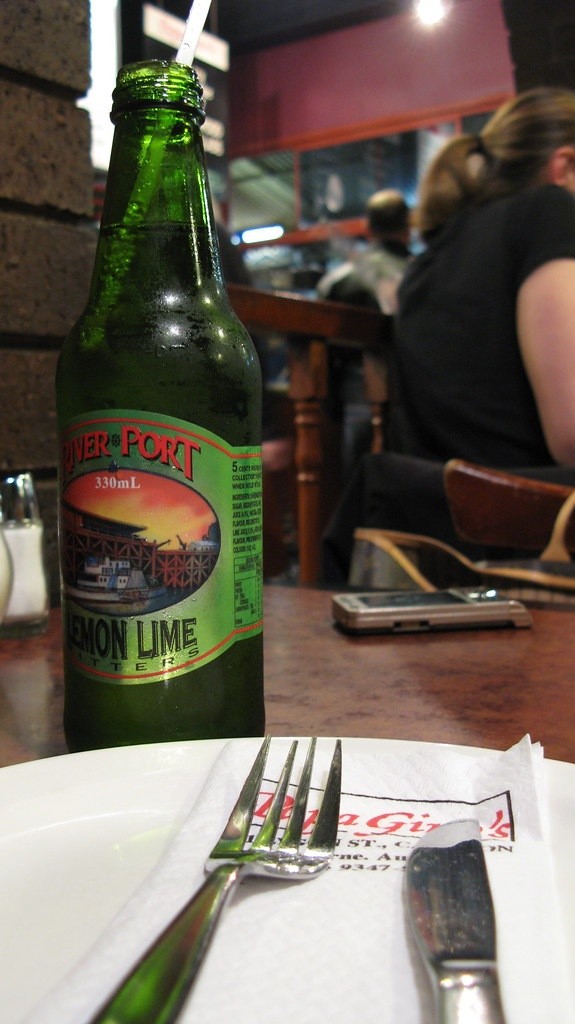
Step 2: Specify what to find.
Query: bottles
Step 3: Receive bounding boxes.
[0,473,48,636]
[57,58,269,745]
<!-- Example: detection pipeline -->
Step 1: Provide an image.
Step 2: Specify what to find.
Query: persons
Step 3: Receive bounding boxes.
[391,83,575,475]
[206,188,435,471]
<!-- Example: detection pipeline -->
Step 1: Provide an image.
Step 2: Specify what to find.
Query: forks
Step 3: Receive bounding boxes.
[84,736,343,1023]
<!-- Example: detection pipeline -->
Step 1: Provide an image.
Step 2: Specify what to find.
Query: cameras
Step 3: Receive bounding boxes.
[332,585,532,633]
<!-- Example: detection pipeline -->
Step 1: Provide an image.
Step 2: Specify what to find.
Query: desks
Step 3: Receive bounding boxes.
[0,584,575,768]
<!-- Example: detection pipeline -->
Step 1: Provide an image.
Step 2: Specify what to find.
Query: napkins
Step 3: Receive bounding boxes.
[23,733,575,1024]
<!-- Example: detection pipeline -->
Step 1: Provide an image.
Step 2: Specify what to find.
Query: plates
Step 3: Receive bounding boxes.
[0,736,575,1024]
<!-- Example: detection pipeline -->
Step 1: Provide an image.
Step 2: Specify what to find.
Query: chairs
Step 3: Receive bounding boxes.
[317,451,575,590]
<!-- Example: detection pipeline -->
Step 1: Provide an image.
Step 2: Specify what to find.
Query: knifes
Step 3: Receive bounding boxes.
[400,817,507,1024]
